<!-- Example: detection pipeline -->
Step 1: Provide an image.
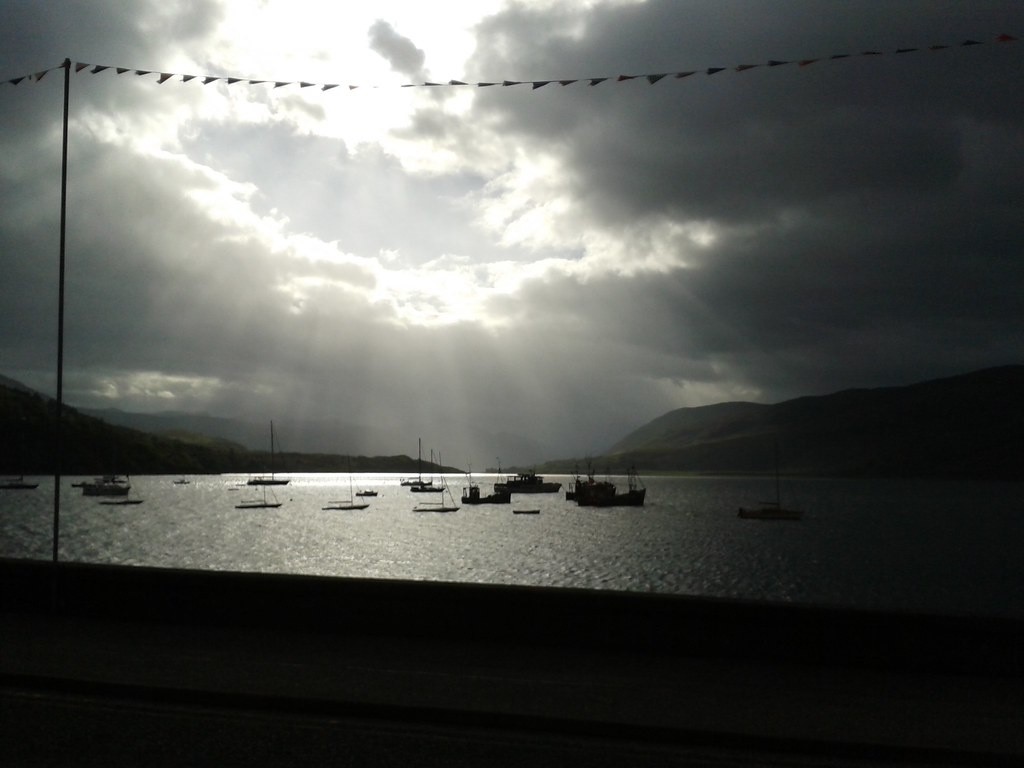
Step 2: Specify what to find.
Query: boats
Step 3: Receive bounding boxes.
[412,476,460,513]
[0,483,40,490]
[566,469,647,507]
[356,490,378,497]
[98,499,145,505]
[321,454,371,510]
[400,437,432,486]
[410,448,446,492]
[494,469,562,493]
[461,482,512,504]
[174,480,189,484]
[234,486,283,509]
[82,473,131,495]
[512,509,541,514]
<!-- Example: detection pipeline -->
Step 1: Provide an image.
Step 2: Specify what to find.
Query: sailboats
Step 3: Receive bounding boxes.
[247,421,291,485]
[737,442,805,520]
